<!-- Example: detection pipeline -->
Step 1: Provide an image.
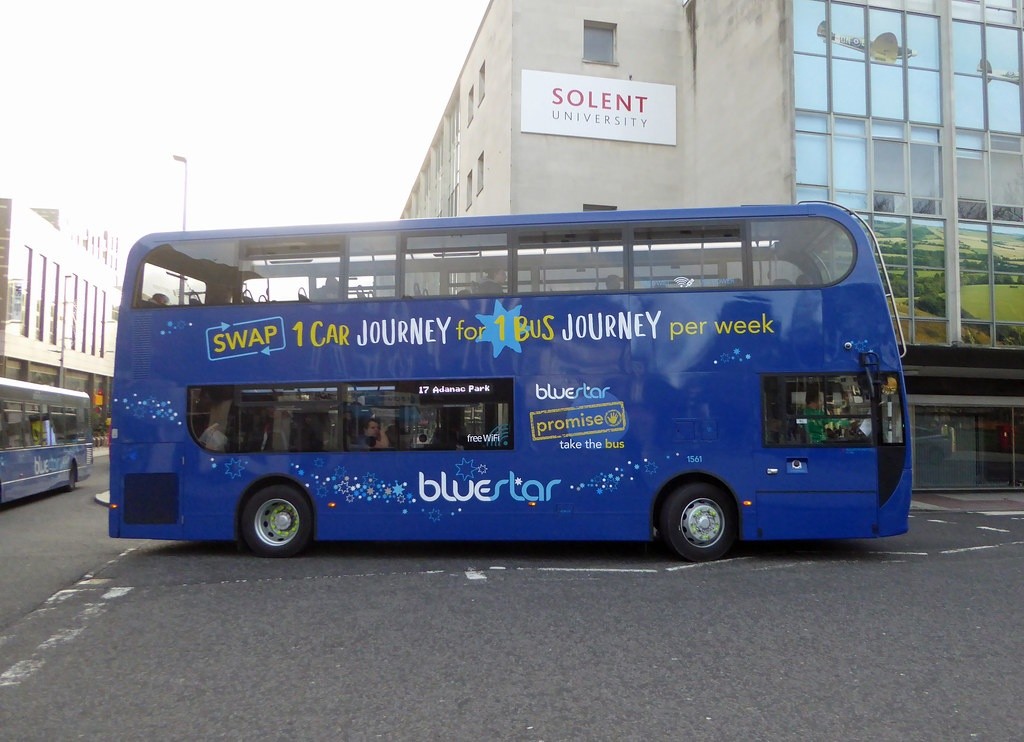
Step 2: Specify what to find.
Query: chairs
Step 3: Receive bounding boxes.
[356,285,367,298]
[298,287,310,301]
[414,282,422,296]
[189,291,204,305]
[242,289,255,303]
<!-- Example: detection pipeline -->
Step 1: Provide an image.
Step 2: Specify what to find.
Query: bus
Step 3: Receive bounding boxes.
[107,199,914,561]
[0,378,93,503]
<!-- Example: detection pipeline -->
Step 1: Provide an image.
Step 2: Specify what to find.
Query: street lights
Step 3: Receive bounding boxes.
[173,154,188,309]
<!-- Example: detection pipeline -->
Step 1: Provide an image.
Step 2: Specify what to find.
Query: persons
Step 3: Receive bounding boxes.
[193,390,338,452]
[803,388,855,443]
[345,387,507,451]
[796,274,814,285]
[606,275,620,290]
[480,267,506,293]
[313,276,339,300]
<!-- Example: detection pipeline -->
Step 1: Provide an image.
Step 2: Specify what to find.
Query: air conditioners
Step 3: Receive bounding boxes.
[417,433,433,444]
